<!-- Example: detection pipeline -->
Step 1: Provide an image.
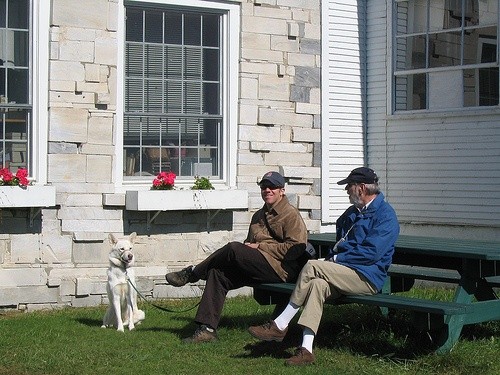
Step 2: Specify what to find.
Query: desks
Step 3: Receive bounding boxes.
[307,233,500,280]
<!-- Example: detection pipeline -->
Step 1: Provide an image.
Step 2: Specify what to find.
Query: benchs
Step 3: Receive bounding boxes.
[247,263,500,360]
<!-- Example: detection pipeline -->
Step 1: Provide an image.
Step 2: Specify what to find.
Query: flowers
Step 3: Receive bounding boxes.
[0,167,29,186]
[153,172,176,190]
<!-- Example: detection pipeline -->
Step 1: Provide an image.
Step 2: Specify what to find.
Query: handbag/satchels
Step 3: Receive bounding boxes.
[295,241,317,267]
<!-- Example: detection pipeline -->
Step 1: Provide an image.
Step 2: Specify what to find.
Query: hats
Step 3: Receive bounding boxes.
[336,166,379,185]
[257,170,285,189]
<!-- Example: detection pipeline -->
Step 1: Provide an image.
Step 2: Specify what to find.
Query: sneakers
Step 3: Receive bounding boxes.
[247,318,288,343]
[166,265,199,286]
[181,326,220,345]
[285,346,315,367]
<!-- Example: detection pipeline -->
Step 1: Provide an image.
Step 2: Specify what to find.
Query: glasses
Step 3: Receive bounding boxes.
[260,184,277,190]
[348,184,357,188]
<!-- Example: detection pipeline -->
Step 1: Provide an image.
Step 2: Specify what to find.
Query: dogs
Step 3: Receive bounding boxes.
[100,231,146,332]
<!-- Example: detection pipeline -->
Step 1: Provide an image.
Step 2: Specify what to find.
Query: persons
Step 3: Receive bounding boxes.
[165,171,308,343]
[248,167,399,365]
